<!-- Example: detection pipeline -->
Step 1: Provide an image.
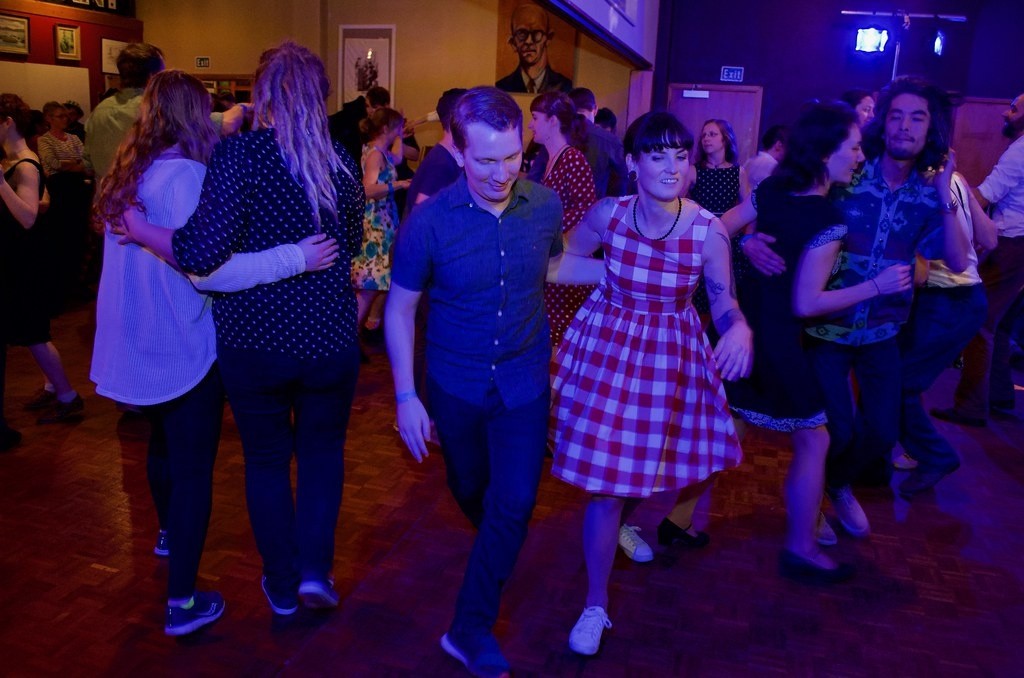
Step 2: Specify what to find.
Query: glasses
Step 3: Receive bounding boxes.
[512,27,548,43]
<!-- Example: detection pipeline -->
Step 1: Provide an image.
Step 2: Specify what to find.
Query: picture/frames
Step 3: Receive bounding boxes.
[101,37,128,74]
[0,13,31,54]
[337,23,396,114]
[55,23,81,60]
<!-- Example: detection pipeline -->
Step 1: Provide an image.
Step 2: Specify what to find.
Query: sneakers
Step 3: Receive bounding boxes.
[826,485,870,537]
[297,574,339,612]
[262,576,298,614]
[164,589,226,636]
[618,523,655,562]
[567,605,613,656]
[815,511,839,546]
[155,529,170,556]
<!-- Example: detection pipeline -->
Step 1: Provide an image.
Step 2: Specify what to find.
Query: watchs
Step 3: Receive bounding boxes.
[940,200,958,213]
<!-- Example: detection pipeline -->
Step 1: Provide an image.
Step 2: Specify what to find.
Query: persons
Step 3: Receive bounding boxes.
[348,87,468,447]
[928,92,1024,428]
[657,77,998,586]
[0,94,104,451]
[526,87,629,459]
[383,85,605,678]
[90,69,339,636]
[563,110,750,655]
[79,42,255,425]
[109,41,366,614]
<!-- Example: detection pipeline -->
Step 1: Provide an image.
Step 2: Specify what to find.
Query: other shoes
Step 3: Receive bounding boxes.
[900,459,960,498]
[438,632,511,678]
[364,325,385,346]
[990,397,1015,409]
[929,407,987,428]
[35,393,84,424]
[892,454,919,469]
[360,347,368,366]
[19,387,57,412]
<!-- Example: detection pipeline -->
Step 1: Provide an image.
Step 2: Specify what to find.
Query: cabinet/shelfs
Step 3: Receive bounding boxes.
[195,74,256,105]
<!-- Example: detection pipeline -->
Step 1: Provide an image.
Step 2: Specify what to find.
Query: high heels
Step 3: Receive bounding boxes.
[776,550,861,586]
[656,517,712,549]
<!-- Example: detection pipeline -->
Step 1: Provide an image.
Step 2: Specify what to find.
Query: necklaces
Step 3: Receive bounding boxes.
[633,196,682,240]
[707,160,725,168]
[544,143,567,180]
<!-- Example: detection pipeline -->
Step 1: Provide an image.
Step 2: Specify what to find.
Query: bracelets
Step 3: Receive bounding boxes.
[872,279,880,295]
[738,234,755,253]
[395,390,417,405]
[387,183,395,196]
[238,104,247,114]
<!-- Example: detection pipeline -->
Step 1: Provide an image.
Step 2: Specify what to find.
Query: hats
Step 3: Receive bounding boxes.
[64,100,85,118]
